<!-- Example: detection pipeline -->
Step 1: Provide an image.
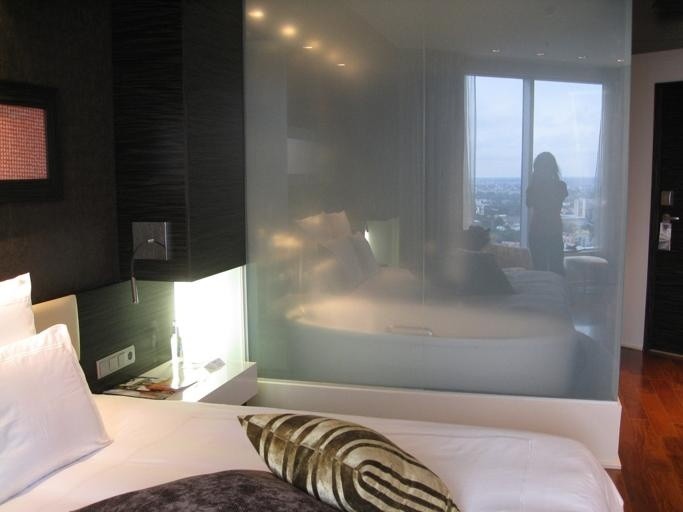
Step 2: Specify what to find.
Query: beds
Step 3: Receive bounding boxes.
[284,267,576,396]
[0,293,625,511]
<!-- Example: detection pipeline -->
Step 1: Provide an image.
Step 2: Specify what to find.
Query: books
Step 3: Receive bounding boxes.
[102,375,197,400]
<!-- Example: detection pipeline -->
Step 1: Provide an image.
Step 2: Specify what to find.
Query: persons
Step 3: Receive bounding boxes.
[525,151,569,275]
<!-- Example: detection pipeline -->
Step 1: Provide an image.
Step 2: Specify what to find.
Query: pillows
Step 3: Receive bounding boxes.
[73,469,338,512]
[290,208,379,295]
[0,270,112,504]
[237,413,459,512]
